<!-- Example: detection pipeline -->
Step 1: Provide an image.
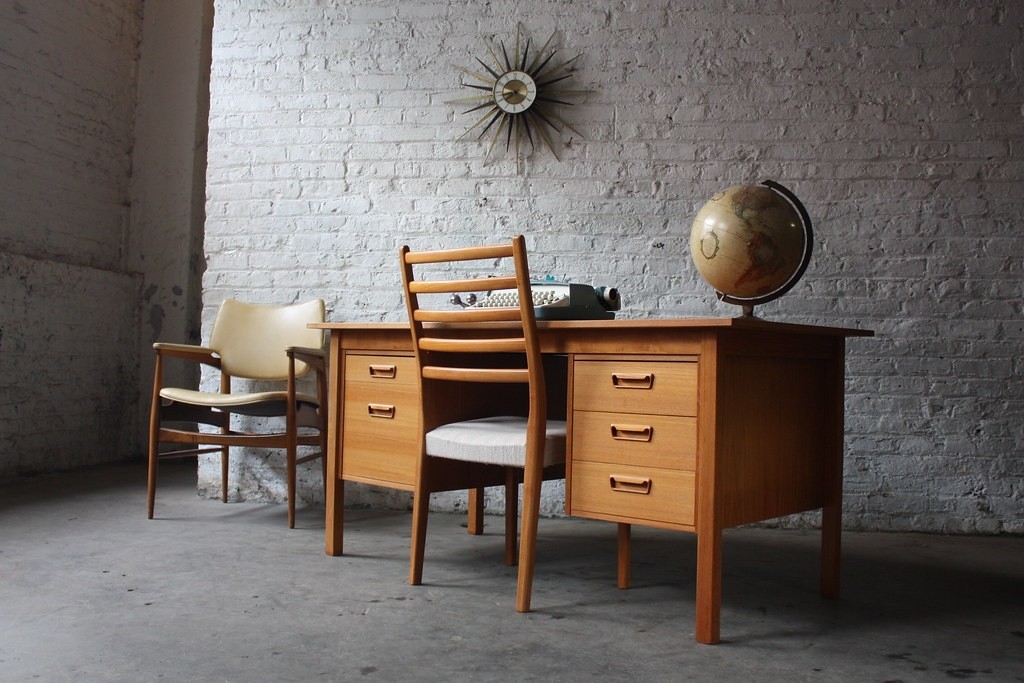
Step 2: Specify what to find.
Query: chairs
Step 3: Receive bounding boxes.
[399,234,630,612]
[146,299,327,530]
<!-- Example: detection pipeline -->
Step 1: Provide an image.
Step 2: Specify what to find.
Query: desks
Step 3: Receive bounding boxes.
[306,317,874,646]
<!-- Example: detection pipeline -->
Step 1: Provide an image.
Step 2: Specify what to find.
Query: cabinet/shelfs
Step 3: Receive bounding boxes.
[341,349,421,490]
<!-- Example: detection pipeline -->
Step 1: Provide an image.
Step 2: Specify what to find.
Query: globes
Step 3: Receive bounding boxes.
[689,178,814,320]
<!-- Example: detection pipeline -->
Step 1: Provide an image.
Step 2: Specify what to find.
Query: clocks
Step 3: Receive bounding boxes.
[443,20,597,176]
